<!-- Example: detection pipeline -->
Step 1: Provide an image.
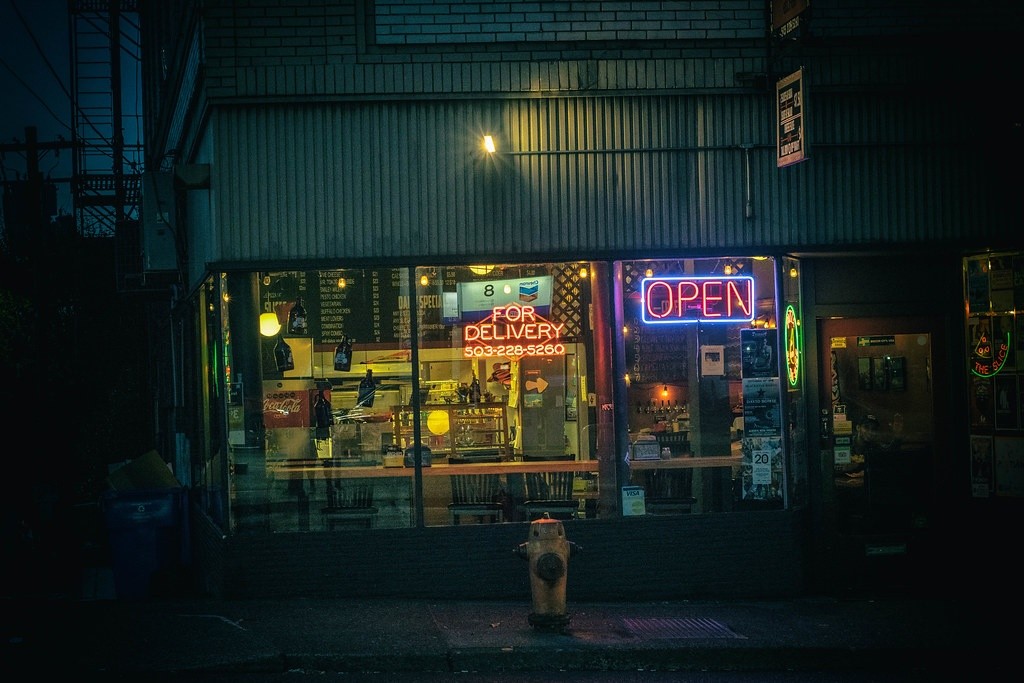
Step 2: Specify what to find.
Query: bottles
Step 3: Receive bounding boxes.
[334,333,353,372]
[629,399,689,423]
[469,377,480,404]
[357,369,375,408]
[273,334,294,371]
[314,390,334,427]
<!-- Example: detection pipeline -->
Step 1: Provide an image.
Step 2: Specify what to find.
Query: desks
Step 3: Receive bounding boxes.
[268,454,741,532]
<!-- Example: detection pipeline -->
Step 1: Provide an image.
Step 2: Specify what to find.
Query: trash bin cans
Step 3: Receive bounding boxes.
[102,490,186,603]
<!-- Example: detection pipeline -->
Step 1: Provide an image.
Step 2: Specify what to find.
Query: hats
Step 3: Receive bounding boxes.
[860,414,880,428]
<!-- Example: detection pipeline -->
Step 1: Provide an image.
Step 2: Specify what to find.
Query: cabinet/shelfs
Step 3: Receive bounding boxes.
[388,401,510,463]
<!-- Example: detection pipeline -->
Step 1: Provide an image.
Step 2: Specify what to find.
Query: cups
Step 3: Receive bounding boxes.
[662,447,671,460]
[673,422,679,432]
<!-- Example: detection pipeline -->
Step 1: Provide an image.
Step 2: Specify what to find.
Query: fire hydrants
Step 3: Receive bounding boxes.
[513,511,585,625]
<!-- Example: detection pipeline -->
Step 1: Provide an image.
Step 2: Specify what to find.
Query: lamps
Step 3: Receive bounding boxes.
[260,292,282,337]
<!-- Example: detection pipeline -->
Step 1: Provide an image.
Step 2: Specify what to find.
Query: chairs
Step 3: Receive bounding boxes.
[320,460,379,531]
[520,454,580,521]
[448,457,504,524]
[637,451,697,515]
[649,430,689,454]
